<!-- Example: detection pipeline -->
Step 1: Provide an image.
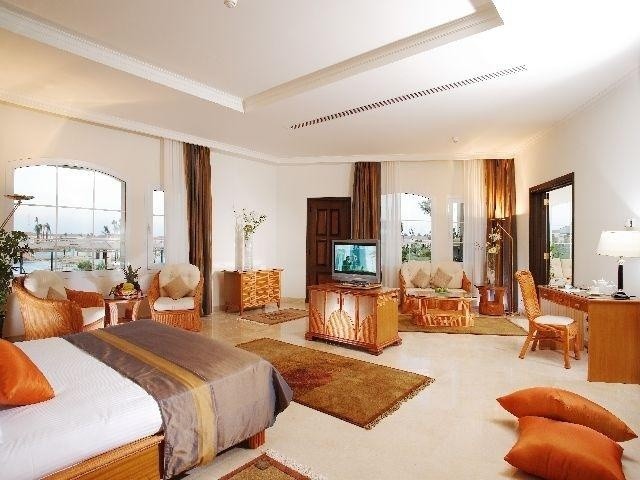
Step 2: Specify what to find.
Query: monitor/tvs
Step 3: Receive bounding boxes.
[331,239,382,284]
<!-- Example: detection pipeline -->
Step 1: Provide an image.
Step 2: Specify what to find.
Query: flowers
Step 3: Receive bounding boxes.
[107,265,143,295]
[472,224,503,270]
[232,209,269,241]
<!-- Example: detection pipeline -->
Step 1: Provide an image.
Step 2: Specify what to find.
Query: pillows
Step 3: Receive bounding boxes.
[0,338,54,407]
[21,271,67,300]
[163,277,192,299]
[410,267,453,288]
[496,387,638,442]
[504,415,627,480]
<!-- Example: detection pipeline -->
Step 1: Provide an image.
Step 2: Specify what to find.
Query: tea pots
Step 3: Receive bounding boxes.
[593,277,614,294]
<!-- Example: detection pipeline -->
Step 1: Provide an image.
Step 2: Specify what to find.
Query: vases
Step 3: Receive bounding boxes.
[245,241,254,270]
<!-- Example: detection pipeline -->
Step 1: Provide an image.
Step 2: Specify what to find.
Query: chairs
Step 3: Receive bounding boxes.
[12,270,105,341]
[514,267,582,369]
[147,264,204,332]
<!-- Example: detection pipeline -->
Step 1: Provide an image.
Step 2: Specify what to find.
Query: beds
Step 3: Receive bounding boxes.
[0,319,294,480]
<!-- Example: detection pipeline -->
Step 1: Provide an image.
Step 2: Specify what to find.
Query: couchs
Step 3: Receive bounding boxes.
[399,260,474,314]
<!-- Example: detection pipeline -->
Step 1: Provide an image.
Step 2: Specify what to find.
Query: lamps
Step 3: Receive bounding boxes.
[0,193,34,230]
[594,229,640,300]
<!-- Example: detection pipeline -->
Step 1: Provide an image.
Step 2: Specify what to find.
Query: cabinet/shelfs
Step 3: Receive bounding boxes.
[222,268,284,316]
[538,284,640,383]
[305,284,402,356]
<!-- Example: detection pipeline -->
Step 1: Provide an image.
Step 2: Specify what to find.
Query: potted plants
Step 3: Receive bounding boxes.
[487,269,496,286]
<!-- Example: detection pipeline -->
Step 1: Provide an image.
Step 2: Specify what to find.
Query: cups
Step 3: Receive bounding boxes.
[590,285,617,296]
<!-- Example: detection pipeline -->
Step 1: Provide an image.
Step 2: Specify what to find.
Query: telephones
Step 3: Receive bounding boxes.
[614,292,629,300]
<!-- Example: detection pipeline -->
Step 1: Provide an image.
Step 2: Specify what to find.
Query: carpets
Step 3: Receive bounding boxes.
[397,315,529,336]
[234,338,436,430]
[218,451,330,480]
[241,307,309,327]
